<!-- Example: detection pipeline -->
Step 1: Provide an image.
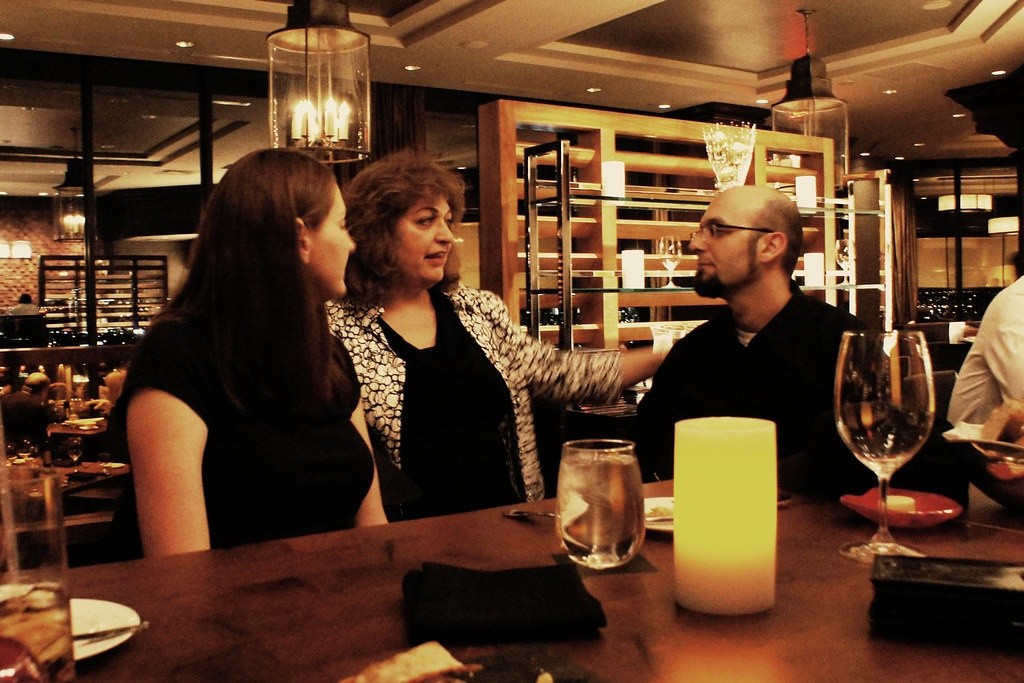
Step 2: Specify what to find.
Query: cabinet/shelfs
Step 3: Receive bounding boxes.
[38,254,170,330]
[525,140,892,350]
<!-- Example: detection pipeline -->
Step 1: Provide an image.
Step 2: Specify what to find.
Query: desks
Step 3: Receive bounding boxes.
[25,460,130,501]
[48,420,108,439]
[0,478,1024,682]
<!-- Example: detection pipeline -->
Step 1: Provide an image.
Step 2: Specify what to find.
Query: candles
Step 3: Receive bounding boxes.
[673,417,777,615]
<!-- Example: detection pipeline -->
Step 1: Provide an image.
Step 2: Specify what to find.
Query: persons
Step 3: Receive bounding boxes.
[10,293,39,315]
[106,145,386,562]
[947,274,1024,428]
[326,154,670,524]
[631,179,882,505]
[0,370,127,465]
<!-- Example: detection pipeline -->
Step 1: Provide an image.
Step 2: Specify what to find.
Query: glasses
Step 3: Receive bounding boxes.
[690,222,776,242]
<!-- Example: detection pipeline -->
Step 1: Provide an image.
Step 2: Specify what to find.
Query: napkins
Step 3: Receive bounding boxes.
[402,560,608,647]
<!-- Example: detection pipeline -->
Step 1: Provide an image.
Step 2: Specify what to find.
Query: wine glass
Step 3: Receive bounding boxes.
[657,236,685,289]
[832,329,935,564]
[66,436,82,472]
[4,437,31,466]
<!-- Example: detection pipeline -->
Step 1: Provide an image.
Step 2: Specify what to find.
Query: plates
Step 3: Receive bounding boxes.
[64,417,105,426]
[62,596,141,660]
[637,498,680,534]
[837,485,963,528]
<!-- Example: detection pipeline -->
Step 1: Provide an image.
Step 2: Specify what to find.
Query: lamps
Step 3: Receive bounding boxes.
[52,127,97,243]
[937,177,1021,237]
[772,9,849,193]
[267,0,373,163]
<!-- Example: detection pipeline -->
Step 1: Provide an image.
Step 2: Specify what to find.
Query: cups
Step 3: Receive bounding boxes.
[555,438,646,569]
[0,465,80,683]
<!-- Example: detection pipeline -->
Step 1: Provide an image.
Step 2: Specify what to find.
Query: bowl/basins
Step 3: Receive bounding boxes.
[942,415,1024,513]
